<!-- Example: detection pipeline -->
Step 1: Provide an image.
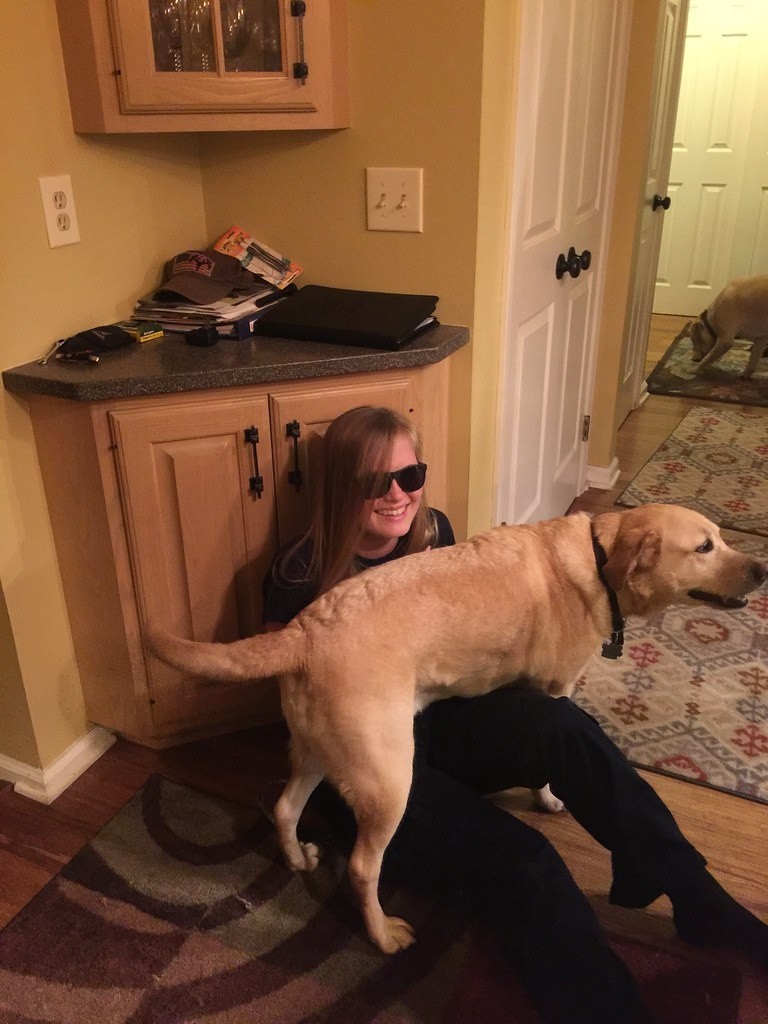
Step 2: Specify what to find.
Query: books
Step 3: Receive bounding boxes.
[259,285,443,350]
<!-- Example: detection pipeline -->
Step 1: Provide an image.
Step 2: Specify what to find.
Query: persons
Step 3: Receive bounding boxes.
[262,404,768,1024]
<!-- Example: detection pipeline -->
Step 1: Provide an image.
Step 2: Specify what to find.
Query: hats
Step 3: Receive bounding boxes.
[153,250,253,305]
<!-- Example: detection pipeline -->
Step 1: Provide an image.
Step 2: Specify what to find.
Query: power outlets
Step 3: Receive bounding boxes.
[38,174,81,250]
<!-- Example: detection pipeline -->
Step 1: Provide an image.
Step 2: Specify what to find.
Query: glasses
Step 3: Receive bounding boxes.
[356,462,427,499]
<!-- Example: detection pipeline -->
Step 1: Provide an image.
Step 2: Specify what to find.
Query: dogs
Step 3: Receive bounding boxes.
[146,501,768,958]
[685,274,767,384]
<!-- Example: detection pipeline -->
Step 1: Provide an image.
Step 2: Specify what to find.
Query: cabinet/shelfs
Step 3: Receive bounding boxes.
[1,321,476,752]
[56,0,353,134]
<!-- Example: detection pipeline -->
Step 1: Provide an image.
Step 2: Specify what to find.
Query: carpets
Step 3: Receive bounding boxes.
[2,771,742,1024]
[613,408,768,536]
[647,321,768,407]
[570,539,767,804]
